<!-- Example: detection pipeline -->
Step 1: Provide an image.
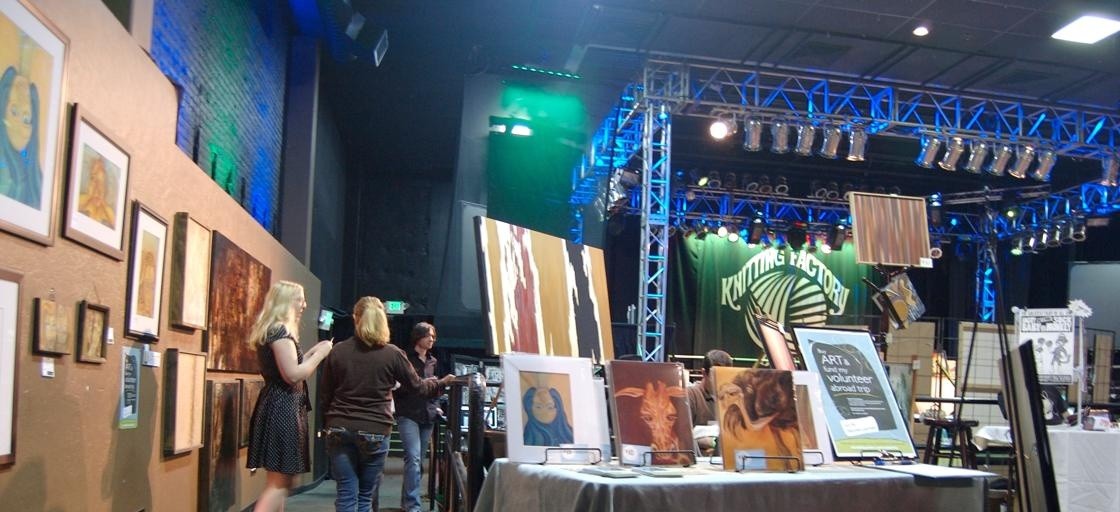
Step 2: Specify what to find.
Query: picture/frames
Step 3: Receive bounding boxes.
[204,231,271,374]
[62,102,131,263]
[501,348,603,466]
[124,199,169,343]
[202,378,241,511]
[0,0,71,246]
[787,320,922,461]
[1,266,27,466]
[168,211,212,331]
[237,378,264,447]
[161,347,207,455]
[34,297,73,356]
[75,301,111,364]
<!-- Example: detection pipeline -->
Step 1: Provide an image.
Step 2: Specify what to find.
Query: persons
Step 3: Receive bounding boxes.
[245,279,334,512]
[686,349,734,457]
[393,320,449,512]
[316,295,457,512]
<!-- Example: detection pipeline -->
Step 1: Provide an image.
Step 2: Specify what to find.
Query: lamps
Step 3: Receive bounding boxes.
[680,212,850,256]
[1009,213,1089,258]
[707,117,1061,185]
[596,166,637,225]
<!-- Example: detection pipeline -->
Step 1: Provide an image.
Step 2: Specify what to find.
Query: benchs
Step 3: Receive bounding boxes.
[914,415,977,468]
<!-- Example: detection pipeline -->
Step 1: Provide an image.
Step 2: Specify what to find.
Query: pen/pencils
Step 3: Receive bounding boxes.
[874,459,917,466]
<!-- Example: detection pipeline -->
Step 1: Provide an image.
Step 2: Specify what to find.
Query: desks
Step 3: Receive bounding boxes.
[1028,419,1120,512]
[458,458,921,512]
